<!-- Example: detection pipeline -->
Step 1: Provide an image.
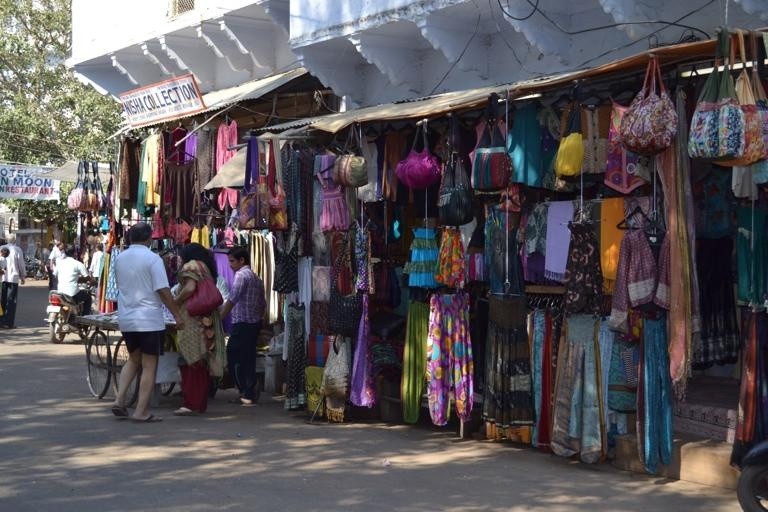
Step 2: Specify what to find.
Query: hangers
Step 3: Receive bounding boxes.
[486,195,530,213]
[162,141,198,164]
[174,215,277,246]
[615,200,667,238]
[169,112,239,134]
[524,294,567,312]
[283,135,358,165]
[487,281,527,302]
[559,210,606,226]
[348,208,379,231]
[643,302,667,322]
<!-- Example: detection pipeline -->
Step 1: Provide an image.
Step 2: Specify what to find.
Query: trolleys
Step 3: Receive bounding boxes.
[73,309,179,413]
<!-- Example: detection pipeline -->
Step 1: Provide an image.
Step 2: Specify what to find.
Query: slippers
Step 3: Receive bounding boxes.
[131,414,162,423]
[111,405,130,417]
[229,397,243,403]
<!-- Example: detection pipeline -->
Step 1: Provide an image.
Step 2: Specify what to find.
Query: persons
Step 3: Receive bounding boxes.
[219,246,266,407]
[110,223,185,422]
[171,241,228,415]
[0,236,104,332]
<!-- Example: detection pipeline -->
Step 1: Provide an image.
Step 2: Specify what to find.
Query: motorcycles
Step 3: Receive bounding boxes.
[47,274,100,344]
[737,441,768,512]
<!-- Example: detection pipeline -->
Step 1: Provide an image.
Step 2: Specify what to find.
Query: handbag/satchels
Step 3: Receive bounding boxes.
[256,325,280,347]
[555,130,585,180]
[620,90,678,157]
[329,292,364,338]
[333,152,369,187]
[469,146,513,191]
[395,149,439,185]
[688,100,768,166]
[65,176,116,212]
[186,278,223,316]
[320,342,349,395]
[438,158,474,226]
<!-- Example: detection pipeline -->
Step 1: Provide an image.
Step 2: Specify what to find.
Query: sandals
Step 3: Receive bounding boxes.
[174,409,206,415]
